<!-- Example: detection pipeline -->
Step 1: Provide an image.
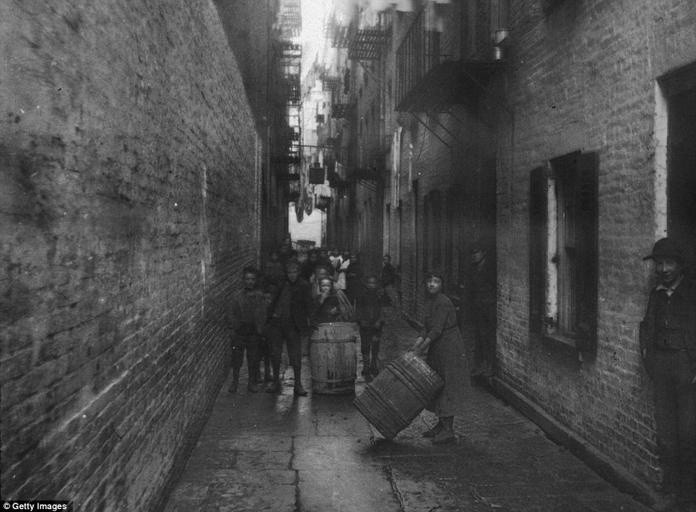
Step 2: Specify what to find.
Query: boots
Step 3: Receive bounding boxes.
[294,367,308,395]
[423,416,455,444]
[229,360,281,393]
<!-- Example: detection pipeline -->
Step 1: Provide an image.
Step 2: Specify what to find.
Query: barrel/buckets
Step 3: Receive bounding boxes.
[351,349,444,441]
[308,322,357,396]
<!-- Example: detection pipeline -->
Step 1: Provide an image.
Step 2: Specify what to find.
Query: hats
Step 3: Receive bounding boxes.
[643,237,686,264]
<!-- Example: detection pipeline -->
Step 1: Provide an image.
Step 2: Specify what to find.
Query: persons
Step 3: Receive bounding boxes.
[226,235,397,396]
[638,236,696,512]
[405,270,473,445]
